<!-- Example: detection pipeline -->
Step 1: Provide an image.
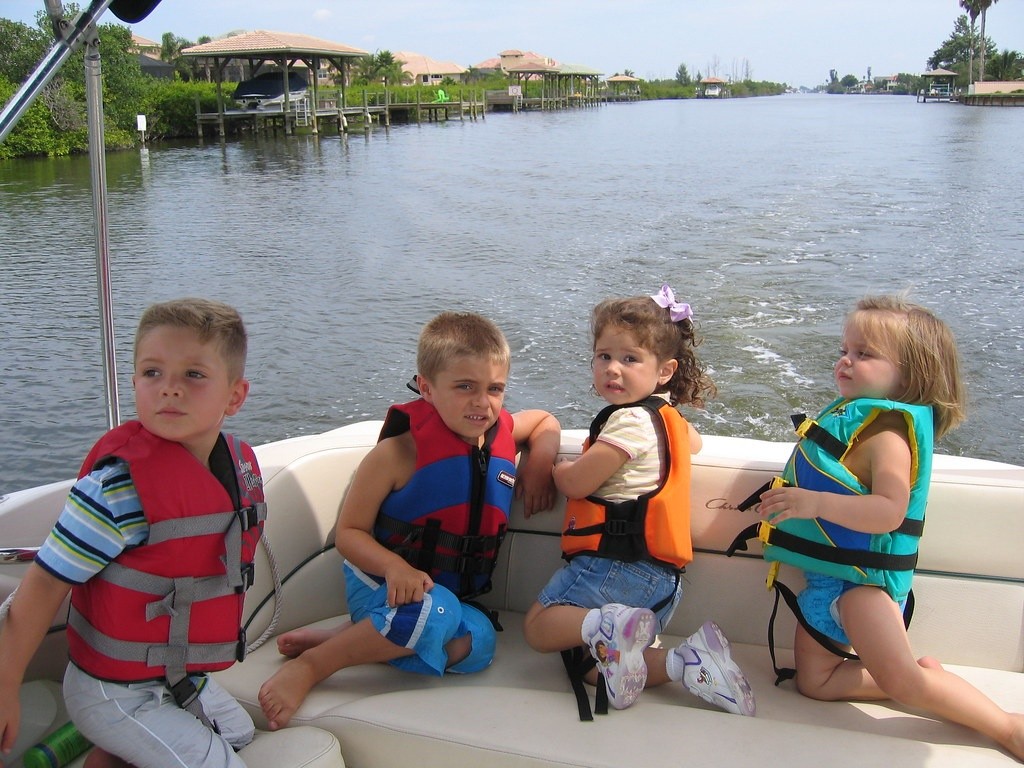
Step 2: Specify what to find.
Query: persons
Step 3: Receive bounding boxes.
[523,297,759,721]
[257,312,562,733]
[0,299,268,767]
[760,297,1024,762]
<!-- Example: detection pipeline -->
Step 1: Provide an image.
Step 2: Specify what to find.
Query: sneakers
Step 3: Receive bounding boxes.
[673,621,756,717]
[588,602,656,710]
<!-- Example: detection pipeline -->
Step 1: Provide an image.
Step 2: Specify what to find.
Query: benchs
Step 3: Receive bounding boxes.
[2,416,1024,767]
